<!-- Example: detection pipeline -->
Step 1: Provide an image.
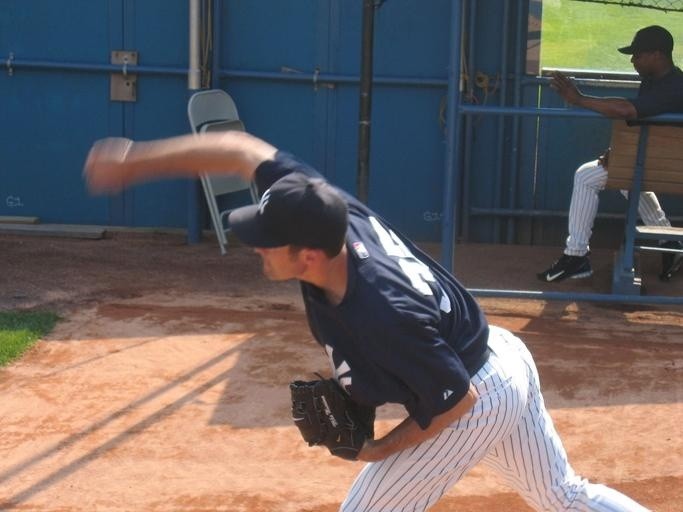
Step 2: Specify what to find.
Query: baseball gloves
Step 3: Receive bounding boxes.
[289,379,375,461]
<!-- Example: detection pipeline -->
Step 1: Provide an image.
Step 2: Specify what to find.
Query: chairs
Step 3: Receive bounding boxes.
[184,90,262,255]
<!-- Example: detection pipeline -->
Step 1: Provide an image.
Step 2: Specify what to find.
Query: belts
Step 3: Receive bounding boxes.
[466,345,492,378]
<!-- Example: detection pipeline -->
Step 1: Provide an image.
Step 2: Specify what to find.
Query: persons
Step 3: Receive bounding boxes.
[81,128,654,512]
[535,25,682,283]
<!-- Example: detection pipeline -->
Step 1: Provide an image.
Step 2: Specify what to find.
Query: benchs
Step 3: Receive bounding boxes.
[603,118,683,296]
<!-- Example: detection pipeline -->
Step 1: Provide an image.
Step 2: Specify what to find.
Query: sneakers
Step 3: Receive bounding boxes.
[658,239,682,280]
[536,250,595,286]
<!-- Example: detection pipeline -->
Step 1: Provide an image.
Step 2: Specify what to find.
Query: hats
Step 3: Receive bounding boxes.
[618,25,674,55]
[228,169,348,252]
